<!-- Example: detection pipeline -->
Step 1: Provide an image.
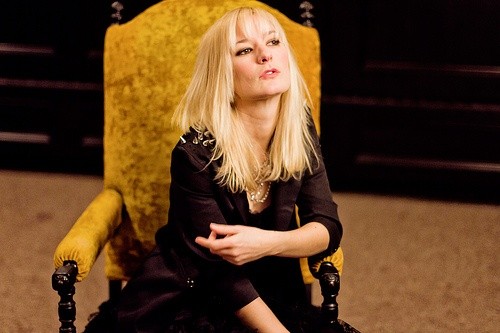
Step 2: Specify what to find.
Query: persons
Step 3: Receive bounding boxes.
[81,6,344,332]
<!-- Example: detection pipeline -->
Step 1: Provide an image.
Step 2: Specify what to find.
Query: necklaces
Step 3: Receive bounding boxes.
[246,149,274,203]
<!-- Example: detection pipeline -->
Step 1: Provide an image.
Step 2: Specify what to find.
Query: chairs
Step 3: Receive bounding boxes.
[46,0,340,333]
